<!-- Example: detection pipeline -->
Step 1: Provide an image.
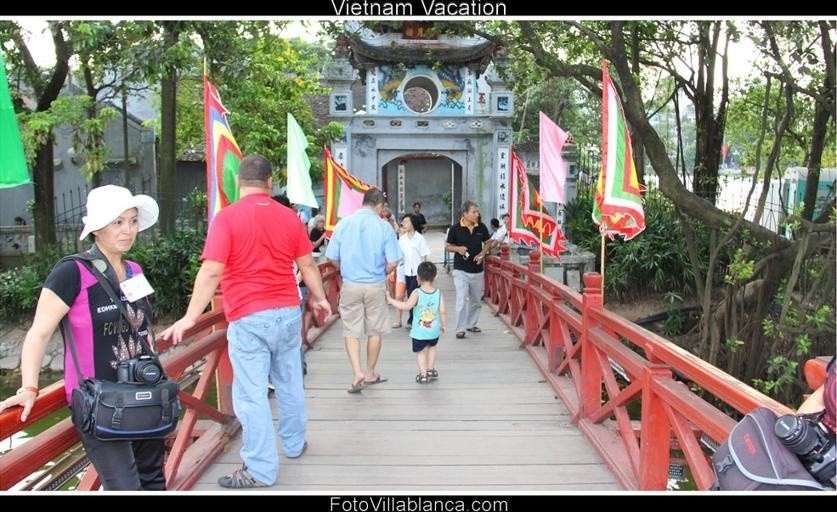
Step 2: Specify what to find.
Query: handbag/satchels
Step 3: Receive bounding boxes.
[61,252,183,442]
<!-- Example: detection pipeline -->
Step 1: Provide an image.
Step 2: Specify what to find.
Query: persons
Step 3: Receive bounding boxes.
[291,203,307,227]
[399,214,430,327]
[392,222,407,328]
[5,189,185,491]
[791,356,837,435]
[445,200,490,339]
[502,213,509,226]
[156,159,331,487]
[327,188,403,394]
[309,215,325,253]
[413,202,428,234]
[387,261,447,384]
[307,206,322,230]
[489,218,508,245]
[269,197,309,392]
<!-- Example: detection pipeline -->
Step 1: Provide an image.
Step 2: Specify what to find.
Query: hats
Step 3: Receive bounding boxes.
[77,184,160,241]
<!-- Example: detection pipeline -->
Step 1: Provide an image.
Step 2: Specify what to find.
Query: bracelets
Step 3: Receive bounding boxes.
[15,388,40,396]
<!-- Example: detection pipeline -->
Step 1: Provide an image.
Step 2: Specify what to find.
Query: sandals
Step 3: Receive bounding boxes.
[415,374,427,384]
[467,326,481,332]
[427,368,439,378]
[456,330,465,338]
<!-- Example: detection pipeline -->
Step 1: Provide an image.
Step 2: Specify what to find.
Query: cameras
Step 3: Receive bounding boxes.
[773,412,837,480]
[118,354,164,386]
[463,251,470,261]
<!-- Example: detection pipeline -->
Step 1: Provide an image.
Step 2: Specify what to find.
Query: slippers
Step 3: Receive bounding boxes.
[347,382,364,393]
[364,375,388,385]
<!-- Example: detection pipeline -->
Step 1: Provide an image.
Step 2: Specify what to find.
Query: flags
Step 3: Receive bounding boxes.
[512,154,566,259]
[326,150,376,240]
[540,110,571,208]
[207,81,241,222]
[594,67,647,239]
[287,112,319,208]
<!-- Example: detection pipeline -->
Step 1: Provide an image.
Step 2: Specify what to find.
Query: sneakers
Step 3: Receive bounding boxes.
[218,463,269,489]
[405,322,411,329]
[286,439,308,459]
[391,320,402,328]
